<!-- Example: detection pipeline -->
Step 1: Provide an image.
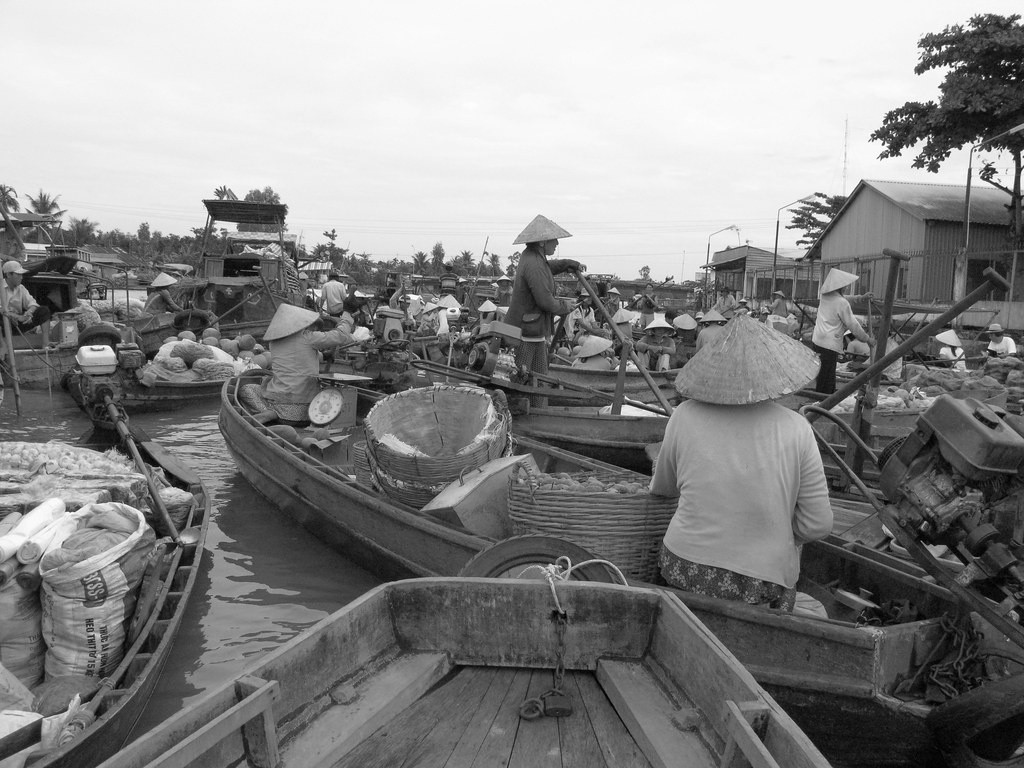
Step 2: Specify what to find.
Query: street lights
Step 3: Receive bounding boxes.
[962,123,1024,250]
[705,224,735,294]
[771,193,823,302]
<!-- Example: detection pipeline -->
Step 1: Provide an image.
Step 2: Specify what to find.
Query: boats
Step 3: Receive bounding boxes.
[0,378,211,768]
[215,366,1024,768]
[0,196,1024,653]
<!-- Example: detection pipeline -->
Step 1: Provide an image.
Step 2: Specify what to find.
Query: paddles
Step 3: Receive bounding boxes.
[446,325,456,382]
[401,279,410,331]
[566,266,674,416]
[721,307,732,315]
[366,301,380,324]
[195,265,280,336]
[866,298,930,387]
[766,306,772,312]
[0,259,22,416]
[323,345,339,373]
[792,299,815,334]
[103,393,183,545]
[806,267,1012,422]
[546,312,566,369]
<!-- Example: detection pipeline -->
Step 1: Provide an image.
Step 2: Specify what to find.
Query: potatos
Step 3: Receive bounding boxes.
[537,472,648,493]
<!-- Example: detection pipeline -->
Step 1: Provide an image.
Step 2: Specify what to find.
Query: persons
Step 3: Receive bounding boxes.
[142,272,184,315]
[505,215,587,406]
[844,328,902,380]
[0,261,52,348]
[650,314,834,611]
[239,294,359,424]
[983,324,1016,359]
[696,288,787,350]
[935,329,965,370]
[564,284,698,372]
[320,274,346,318]
[388,282,461,336]
[813,270,876,393]
[458,275,513,324]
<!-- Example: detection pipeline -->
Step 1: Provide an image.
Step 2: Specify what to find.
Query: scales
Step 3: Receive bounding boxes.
[304,372,373,433]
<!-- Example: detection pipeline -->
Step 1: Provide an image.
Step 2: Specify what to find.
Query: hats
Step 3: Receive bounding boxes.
[820,267,860,294]
[437,294,462,308]
[151,271,177,287]
[612,307,635,324]
[644,318,675,336]
[459,278,467,282]
[577,335,613,358]
[985,324,1004,333]
[695,285,962,347]
[607,287,621,295]
[674,312,698,330]
[3,261,29,274]
[491,282,499,288]
[674,311,822,404]
[512,215,572,244]
[496,275,511,283]
[263,303,319,340]
[478,299,497,311]
[422,302,439,314]
[298,271,309,279]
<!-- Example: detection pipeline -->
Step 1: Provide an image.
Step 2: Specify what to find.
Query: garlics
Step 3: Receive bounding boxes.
[0,440,130,475]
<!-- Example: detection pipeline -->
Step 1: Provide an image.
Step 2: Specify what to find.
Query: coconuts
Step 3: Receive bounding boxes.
[163,328,271,369]
